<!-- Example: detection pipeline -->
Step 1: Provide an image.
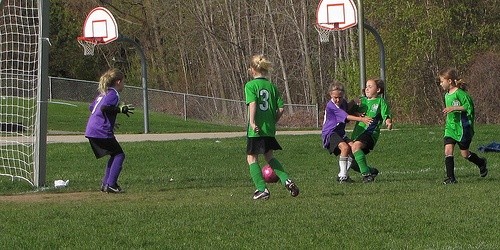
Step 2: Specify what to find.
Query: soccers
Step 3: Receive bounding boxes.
[262,164,280,183]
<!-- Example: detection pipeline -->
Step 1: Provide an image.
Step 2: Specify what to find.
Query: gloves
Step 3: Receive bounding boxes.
[121,104,135,117]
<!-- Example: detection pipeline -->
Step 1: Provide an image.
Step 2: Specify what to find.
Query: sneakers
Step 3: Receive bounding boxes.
[285,178,300,197]
[252,188,271,200]
[337,175,349,182]
[442,176,456,184]
[363,176,374,183]
[478,157,488,177]
[100,183,125,193]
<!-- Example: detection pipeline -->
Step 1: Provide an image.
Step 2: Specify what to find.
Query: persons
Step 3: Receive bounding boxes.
[84,69,135,193]
[321,81,374,184]
[244,55,299,200]
[350,79,392,183]
[437,68,487,183]
